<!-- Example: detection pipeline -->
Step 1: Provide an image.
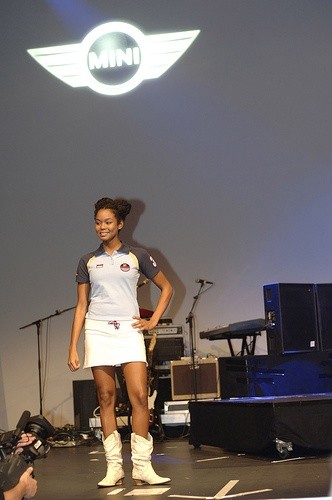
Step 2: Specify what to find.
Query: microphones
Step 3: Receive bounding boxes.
[15,410,32,438]
[196,278,212,284]
[136,279,150,289]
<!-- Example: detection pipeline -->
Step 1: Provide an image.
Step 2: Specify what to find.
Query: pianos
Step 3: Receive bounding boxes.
[200,319,266,357]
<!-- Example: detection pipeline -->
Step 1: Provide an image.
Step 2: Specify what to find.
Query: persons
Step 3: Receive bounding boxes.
[0,433,37,500]
[68,198,173,487]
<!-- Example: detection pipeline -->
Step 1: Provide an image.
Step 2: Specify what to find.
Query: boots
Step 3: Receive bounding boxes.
[130,431,172,486]
[97,430,125,486]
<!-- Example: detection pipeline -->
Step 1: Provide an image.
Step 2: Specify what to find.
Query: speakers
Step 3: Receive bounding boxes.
[170,359,221,401]
[263,282,332,355]
